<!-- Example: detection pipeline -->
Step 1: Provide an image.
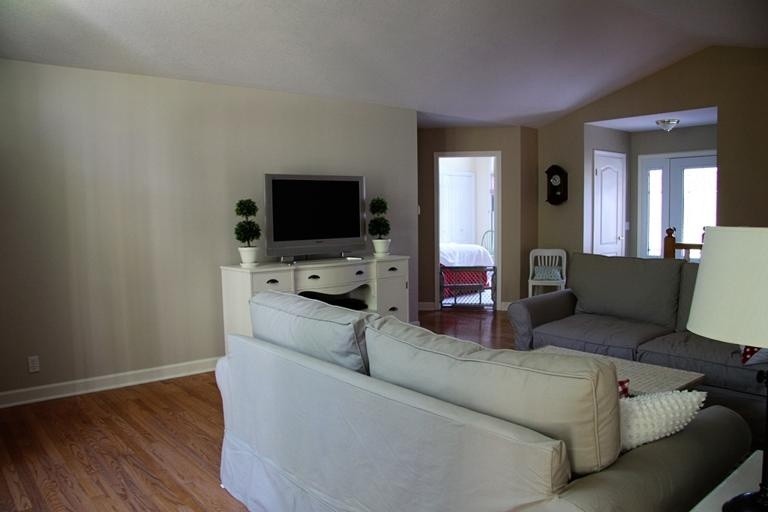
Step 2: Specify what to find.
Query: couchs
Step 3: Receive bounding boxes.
[214,288,752,512]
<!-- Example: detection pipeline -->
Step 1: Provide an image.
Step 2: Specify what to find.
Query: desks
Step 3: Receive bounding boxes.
[508,252,768,434]
[691,450,763,512]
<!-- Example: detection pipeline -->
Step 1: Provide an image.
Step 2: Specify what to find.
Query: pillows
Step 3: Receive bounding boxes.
[363,315,621,475]
[569,254,681,329]
[676,263,699,332]
[247,288,379,376]
[534,266,561,280]
[617,390,707,450]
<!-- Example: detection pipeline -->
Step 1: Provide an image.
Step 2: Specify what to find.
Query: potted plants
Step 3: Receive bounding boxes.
[233,198,261,262]
[367,197,391,253]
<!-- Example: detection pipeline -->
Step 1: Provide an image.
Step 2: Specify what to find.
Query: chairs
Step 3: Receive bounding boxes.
[528,249,567,297]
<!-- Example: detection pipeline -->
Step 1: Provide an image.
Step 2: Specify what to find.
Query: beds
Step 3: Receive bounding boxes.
[439,243,495,297]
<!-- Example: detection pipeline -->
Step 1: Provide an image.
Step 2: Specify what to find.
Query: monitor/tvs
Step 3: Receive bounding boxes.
[265,173,366,266]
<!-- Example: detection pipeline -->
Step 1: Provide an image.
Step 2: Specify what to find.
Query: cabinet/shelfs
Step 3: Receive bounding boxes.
[221,254,411,338]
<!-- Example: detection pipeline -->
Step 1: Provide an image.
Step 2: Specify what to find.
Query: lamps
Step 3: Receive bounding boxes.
[657,119,678,131]
[686,226,767,352]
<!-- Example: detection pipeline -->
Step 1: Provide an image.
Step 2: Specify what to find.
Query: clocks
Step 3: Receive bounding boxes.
[545,165,568,206]
